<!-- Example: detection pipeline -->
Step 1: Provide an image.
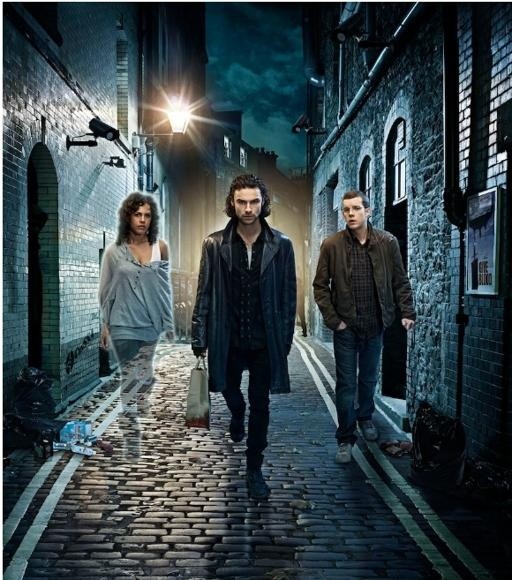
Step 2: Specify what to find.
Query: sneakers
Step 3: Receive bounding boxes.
[335,442,352,463]
[358,417,379,442]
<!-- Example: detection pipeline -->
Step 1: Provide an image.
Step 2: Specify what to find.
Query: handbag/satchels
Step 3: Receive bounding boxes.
[185,356,211,428]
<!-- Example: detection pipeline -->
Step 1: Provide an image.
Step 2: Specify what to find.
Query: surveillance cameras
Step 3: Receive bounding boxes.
[291,114,308,134]
[89,118,119,141]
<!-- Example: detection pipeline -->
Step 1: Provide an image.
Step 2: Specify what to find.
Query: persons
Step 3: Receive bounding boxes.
[96,193,175,463]
[311,189,417,465]
[189,174,298,505]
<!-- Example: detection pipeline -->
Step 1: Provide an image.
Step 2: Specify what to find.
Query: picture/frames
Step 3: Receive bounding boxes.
[464,187,501,297]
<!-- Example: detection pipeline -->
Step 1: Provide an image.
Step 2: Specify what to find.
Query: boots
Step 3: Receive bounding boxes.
[245,452,270,503]
[228,403,246,442]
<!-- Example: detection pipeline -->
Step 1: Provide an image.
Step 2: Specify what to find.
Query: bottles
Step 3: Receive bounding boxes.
[71,445,96,456]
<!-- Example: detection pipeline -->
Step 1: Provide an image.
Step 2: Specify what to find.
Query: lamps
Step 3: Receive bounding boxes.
[66,97,192,172]
